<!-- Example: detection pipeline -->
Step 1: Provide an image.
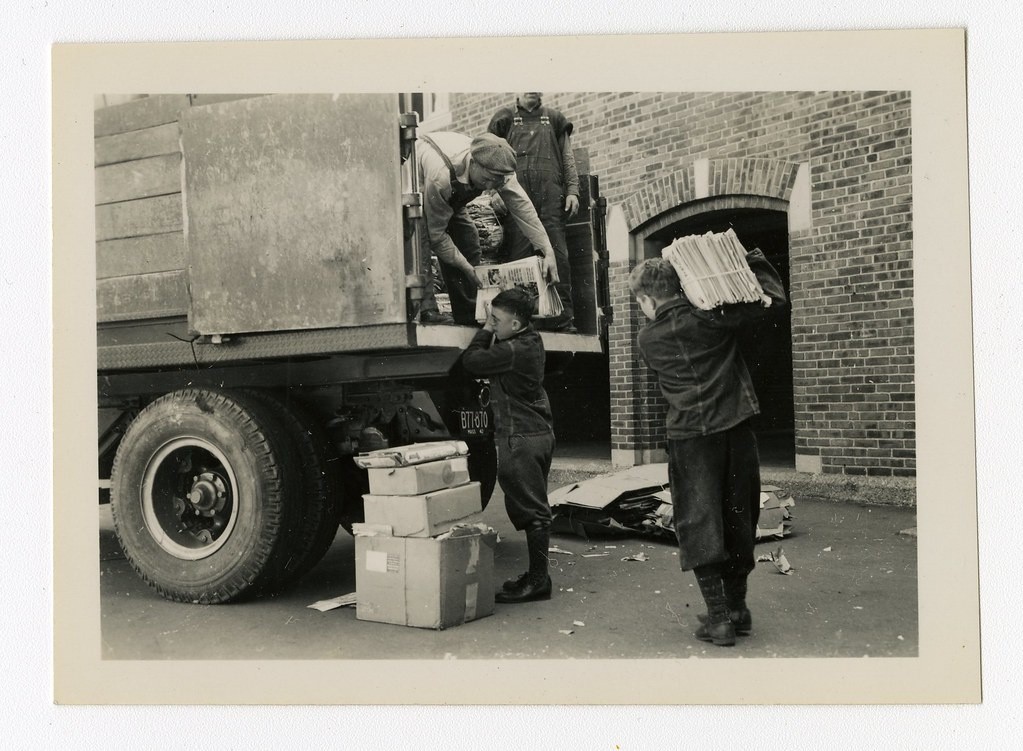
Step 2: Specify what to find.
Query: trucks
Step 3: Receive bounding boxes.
[95,93,605,606]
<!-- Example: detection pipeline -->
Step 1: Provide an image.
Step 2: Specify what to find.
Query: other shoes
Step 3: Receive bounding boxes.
[561,322,577,334]
[420,309,454,325]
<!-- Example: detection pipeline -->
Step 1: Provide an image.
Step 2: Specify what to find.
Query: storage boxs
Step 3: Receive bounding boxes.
[354,440,469,469]
[360,482,483,538]
[356,532,499,629]
[370,457,471,495]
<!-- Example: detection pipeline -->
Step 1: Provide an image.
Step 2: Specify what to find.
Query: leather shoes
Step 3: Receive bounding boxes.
[697,608,751,630]
[495,576,551,603]
[696,618,735,645]
[503,572,552,593]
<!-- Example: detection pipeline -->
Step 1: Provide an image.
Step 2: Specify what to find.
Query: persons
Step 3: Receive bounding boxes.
[460,288,557,604]
[486,92,581,334]
[628,242,786,647]
[413,130,566,325]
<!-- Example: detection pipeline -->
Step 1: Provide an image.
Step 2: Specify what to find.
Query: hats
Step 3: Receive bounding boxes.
[470,133,517,175]
